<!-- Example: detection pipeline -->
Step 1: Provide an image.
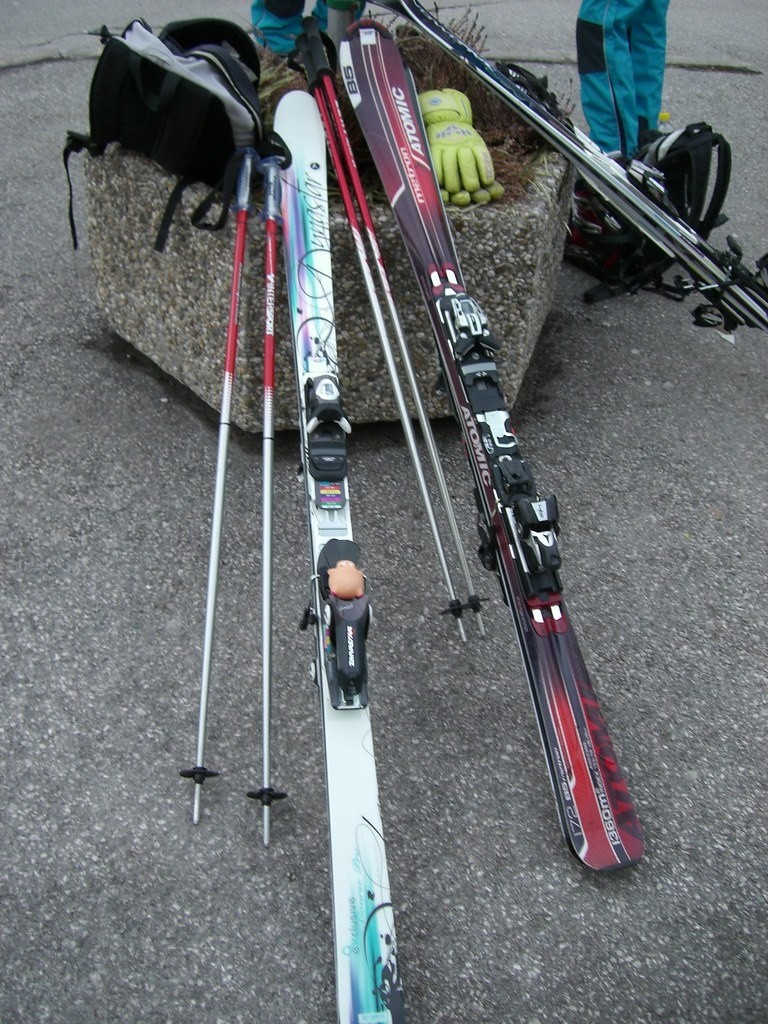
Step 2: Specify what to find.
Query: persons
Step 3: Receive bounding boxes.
[248,0,367,73]
[574,0,669,161]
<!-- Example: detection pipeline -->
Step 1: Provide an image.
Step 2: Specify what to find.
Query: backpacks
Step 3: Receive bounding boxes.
[63,16,292,252]
[563,123,731,305]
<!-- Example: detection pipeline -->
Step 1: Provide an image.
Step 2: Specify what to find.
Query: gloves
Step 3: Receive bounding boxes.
[418,87,506,206]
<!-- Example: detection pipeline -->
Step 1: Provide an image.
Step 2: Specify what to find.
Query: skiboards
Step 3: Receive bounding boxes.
[390,0,768,328]
[276,32,641,1024]
